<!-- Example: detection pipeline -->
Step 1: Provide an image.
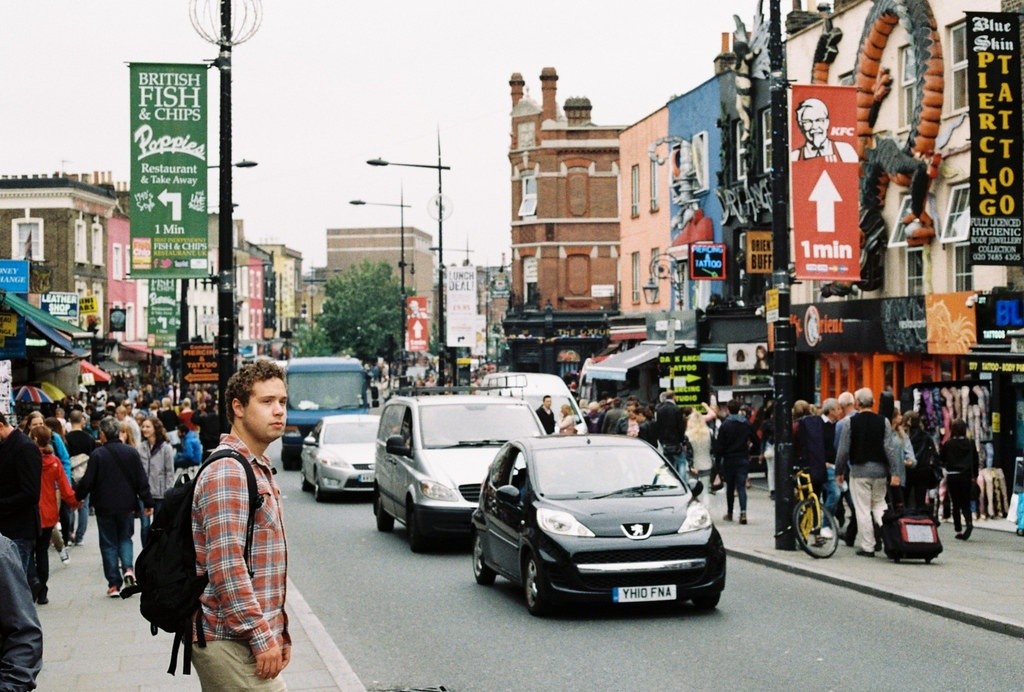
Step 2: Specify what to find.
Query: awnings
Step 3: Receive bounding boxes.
[127,344,171,358]
[79,361,111,381]
[0,292,94,371]
[586,345,682,381]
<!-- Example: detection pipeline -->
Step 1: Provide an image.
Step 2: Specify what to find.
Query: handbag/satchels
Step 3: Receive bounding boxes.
[941,495,952,518]
[662,443,682,456]
[709,456,724,491]
[70,454,89,484]
[970,483,980,500]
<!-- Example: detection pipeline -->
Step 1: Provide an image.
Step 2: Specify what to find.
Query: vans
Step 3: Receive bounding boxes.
[372,393,549,554]
[479,371,590,436]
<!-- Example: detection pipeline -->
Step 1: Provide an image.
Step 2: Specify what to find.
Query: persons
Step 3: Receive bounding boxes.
[471,376,482,395]
[364,362,397,383]
[558,405,575,435]
[0,373,219,603]
[791,387,979,557]
[415,363,453,395]
[535,395,556,435]
[189,361,291,692]
[755,345,769,369]
[579,390,773,524]
[564,371,580,395]
[0,532,43,692]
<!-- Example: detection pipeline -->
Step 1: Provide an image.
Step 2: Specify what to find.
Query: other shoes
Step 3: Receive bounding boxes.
[955,534,963,540]
[964,524,974,540]
[75,538,83,546]
[64,536,75,547]
[29,577,41,602]
[810,527,820,535]
[856,550,874,558]
[122,569,136,587]
[107,585,120,597]
[836,512,846,528]
[723,514,733,521]
[59,544,70,566]
[739,513,747,524]
[820,528,833,540]
[36,594,49,605]
[837,530,854,548]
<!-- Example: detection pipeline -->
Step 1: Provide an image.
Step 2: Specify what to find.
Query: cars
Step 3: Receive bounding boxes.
[468,434,729,619]
[299,413,382,503]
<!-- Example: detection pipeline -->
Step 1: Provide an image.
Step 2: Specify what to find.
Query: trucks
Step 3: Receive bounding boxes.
[281,357,379,480]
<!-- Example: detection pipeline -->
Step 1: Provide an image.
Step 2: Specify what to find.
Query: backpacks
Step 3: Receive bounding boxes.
[120,449,258,678]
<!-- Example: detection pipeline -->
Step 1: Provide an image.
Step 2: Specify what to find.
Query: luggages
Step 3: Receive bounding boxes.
[882,483,943,564]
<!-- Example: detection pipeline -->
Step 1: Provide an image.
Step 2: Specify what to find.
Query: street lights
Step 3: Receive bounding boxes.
[365,123,446,396]
[177,160,259,401]
[348,181,409,390]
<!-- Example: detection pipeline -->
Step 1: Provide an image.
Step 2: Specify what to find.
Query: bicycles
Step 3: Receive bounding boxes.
[791,465,839,558]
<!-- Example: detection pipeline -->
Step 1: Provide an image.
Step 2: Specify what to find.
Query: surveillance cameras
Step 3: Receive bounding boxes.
[965,300,975,308]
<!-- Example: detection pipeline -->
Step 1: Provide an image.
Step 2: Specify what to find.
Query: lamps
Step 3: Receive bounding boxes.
[642,253,684,313]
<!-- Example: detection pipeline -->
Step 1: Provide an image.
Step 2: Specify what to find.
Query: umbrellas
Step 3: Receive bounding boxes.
[13,386,53,404]
[30,381,65,400]
